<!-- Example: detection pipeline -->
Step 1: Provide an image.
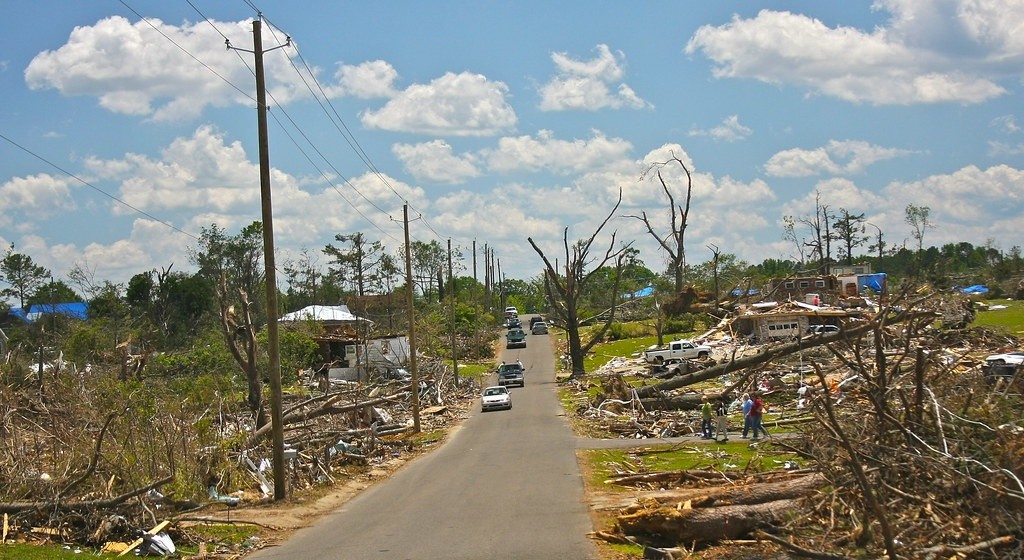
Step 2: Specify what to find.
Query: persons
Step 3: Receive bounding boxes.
[711,395,729,440]
[740,392,767,439]
[814,295,818,306]
[700,395,712,438]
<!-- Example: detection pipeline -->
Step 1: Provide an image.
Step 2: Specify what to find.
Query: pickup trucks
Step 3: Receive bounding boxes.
[645,340,711,365]
[497,363,525,387]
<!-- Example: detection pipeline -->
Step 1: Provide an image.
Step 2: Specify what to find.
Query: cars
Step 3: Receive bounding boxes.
[528,317,549,335]
[507,318,523,330]
[505,330,527,349]
[505,306,518,317]
[480,386,512,411]
[806,324,841,338]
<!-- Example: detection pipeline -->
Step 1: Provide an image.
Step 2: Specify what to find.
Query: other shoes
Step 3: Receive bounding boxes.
[750,437,758,441]
[761,435,768,439]
[739,435,747,438]
[722,438,729,441]
[700,436,712,439]
[711,437,718,441]
